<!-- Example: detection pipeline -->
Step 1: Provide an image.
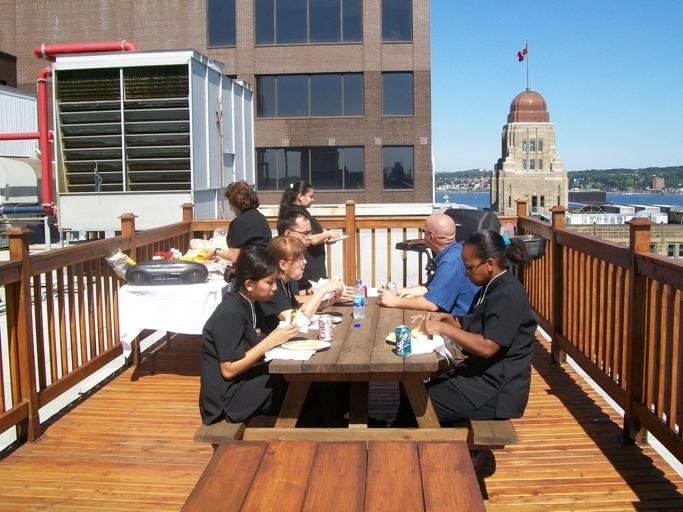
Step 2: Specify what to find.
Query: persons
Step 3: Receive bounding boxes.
[199,243,363,427]
[277,182,340,288]
[255,237,389,427]
[392,230,537,430]
[378,212,481,316]
[199,181,272,263]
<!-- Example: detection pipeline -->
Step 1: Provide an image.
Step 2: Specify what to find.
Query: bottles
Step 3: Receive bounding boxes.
[353,279,366,320]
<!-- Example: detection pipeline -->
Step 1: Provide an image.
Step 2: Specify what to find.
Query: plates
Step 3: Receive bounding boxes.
[309,314,342,324]
[281,340,331,352]
[325,235,349,243]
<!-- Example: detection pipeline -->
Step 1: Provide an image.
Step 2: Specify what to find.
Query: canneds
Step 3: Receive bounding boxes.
[357,286,369,306]
[396,326,412,357]
[386,282,398,296]
[319,314,334,341]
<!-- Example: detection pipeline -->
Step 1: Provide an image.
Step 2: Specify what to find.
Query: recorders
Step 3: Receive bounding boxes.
[125,260,208,284]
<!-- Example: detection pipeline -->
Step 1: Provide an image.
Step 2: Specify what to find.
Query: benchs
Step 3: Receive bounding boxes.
[194,418,247,448]
[470,414,519,467]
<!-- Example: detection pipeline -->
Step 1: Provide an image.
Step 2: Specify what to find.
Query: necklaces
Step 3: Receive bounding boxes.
[477,270,508,307]
[239,291,257,330]
[280,278,291,299]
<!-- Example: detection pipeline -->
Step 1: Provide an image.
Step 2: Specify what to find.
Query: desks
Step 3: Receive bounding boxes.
[118,277,233,375]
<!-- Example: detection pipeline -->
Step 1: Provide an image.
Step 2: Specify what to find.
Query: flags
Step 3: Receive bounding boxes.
[517,46,528,62]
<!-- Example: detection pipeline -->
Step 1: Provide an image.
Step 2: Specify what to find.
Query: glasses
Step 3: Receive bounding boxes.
[466,260,485,274]
[290,229,311,236]
[288,255,304,262]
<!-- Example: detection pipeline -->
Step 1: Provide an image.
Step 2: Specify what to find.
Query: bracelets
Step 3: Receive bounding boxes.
[214,247,221,253]
